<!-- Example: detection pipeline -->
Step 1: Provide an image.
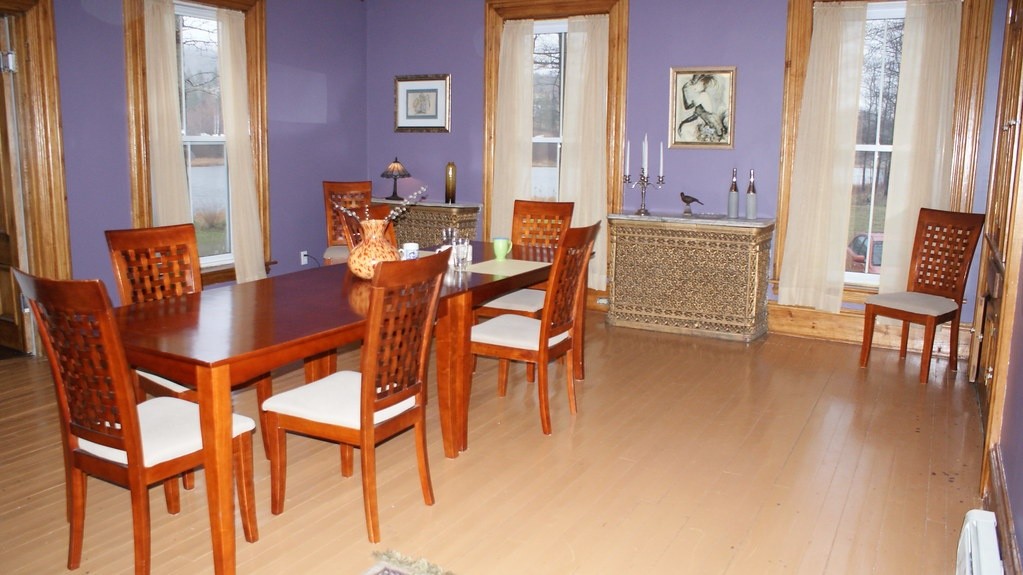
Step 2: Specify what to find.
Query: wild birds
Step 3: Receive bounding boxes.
[680,192,704,206]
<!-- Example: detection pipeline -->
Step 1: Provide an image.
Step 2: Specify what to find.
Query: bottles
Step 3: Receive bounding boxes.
[441,227,473,272]
[746,169,757,220]
[727,168,739,219]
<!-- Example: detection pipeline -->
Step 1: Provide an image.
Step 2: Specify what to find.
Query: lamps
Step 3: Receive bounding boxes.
[381,155,412,201]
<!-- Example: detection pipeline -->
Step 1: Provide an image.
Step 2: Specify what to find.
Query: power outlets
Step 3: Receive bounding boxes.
[301,251,308,265]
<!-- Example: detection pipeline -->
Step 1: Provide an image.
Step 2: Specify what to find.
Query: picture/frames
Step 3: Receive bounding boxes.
[393,73,452,134]
[668,67,736,150]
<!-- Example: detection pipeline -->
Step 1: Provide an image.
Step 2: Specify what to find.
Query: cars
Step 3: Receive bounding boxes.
[846,232,883,274]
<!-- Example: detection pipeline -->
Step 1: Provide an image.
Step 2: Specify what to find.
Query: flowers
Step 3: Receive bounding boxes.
[329,185,426,221]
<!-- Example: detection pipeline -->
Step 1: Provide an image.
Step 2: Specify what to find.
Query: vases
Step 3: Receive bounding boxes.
[347,219,400,279]
[445,161,457,203]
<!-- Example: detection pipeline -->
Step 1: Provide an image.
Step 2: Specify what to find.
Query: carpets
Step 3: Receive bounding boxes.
[367,551,452,574]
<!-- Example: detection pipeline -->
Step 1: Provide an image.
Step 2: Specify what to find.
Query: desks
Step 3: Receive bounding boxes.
[64,240,595,575]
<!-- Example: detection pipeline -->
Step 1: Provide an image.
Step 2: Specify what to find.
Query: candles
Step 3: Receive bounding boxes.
[626,140,630,176]
[659,142,663,177]
[641,133,648,177]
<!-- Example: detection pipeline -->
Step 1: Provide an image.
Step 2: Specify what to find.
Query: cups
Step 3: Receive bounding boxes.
[493,237,512,261]
[435,244,455,266]
[398,243,419,261]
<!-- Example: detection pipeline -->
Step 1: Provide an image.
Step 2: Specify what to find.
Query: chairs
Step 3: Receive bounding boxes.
[859,207,986,384]
[304,182,397,389]
[262,248,453,544]
[105,221,274,489]
[10,264,259,575]
[465,198,600,437]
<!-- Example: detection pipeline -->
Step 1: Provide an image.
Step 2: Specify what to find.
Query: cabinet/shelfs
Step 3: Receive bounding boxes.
[968,1,1023,497]
[606,211,776,344]
[371,199,481,249]
